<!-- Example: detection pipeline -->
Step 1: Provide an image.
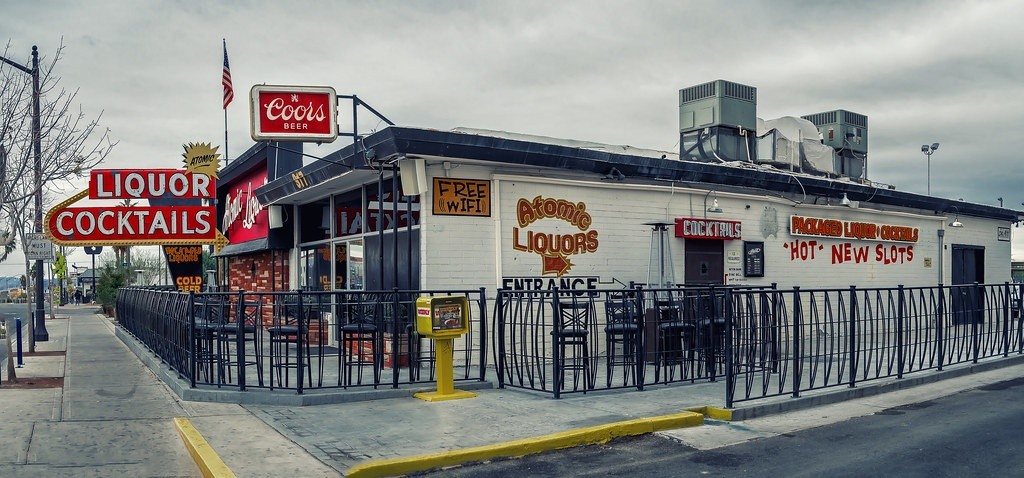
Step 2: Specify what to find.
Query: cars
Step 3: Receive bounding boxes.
[1010,285,1021,315]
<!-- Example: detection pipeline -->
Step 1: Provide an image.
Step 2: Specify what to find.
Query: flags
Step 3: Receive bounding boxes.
[221,40,234,110]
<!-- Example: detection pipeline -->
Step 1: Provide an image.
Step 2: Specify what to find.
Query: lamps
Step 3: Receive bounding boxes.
[839,192,851,204]
[941,206,964,228]
[704,189,723,219]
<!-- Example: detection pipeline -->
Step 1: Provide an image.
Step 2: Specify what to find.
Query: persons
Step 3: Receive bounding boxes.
[74,289,81,306]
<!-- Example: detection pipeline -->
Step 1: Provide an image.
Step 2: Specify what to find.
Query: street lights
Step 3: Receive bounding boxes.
[921,143,939,195]
[998,197,1003,207]
[6,274,24,299]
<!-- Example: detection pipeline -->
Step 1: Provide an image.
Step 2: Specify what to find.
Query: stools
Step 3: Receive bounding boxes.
[265,301,314,390]
[193,298,263,390]
[338,298,379,389]
[406,296,437,382]
[605,300,640,387]
[698,294,727,377]
[549,300,591,390]
[655,300,695,384]
